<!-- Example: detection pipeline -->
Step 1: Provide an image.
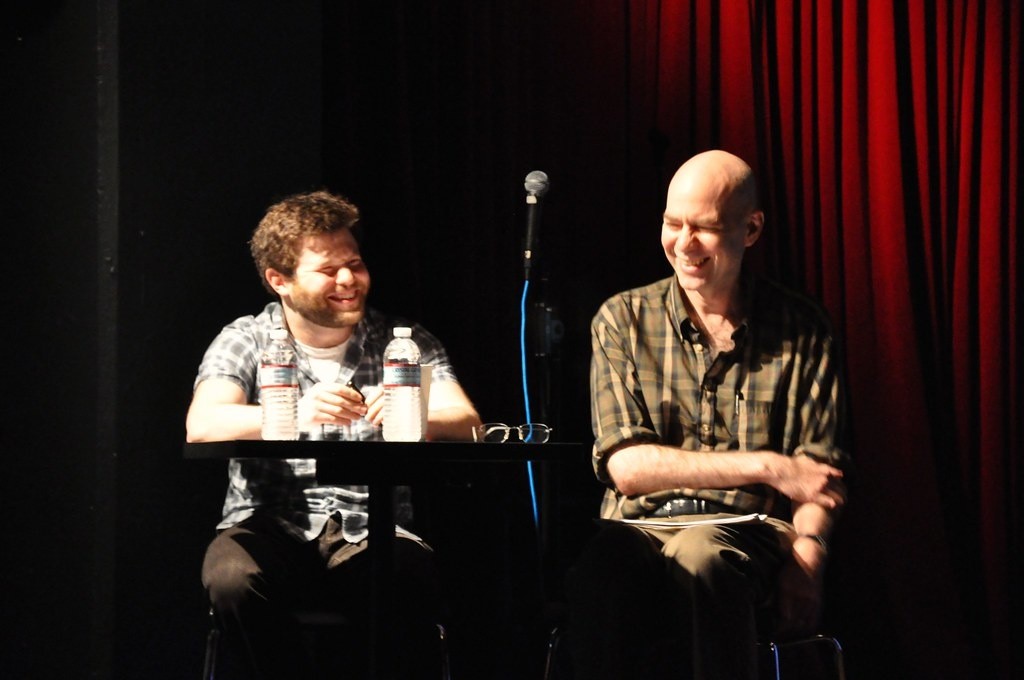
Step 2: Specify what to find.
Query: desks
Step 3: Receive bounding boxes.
[182,439,586,680]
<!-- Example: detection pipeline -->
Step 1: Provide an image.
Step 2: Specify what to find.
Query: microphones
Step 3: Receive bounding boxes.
[523,171,550,280]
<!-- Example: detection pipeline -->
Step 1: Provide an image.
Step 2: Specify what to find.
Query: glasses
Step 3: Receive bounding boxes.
[471,423,553,444]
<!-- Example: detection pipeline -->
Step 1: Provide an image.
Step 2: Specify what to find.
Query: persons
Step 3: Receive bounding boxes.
[186,192,484,680]
[561,151,851,680]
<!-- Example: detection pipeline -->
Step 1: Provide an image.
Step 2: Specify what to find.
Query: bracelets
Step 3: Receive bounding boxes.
[806,534,826,548]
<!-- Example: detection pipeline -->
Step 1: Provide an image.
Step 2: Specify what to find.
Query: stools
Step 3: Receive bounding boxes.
[201,596,452,680]
[542,623,846,680]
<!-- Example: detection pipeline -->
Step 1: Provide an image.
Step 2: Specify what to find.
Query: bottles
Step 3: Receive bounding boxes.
[383,327,423,442]
[260,330,301,440]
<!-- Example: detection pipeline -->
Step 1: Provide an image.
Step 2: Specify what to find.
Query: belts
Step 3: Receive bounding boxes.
[645,497,736,516]
